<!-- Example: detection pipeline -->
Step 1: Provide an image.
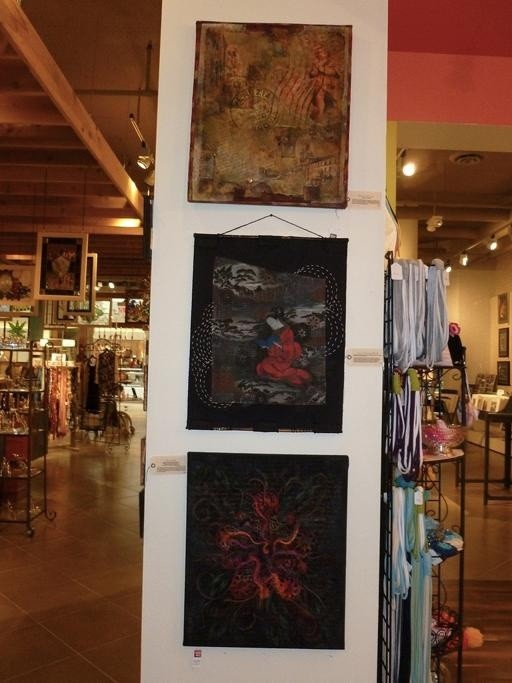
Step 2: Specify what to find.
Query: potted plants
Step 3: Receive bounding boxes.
[8,320,27,346]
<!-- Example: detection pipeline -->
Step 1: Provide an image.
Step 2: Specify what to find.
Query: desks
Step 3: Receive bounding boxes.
[456,410,512,506]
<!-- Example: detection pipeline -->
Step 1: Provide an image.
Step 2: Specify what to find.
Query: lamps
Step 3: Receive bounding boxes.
[444,218,512,272]
[129,114,155,187]
[397,147,414,176]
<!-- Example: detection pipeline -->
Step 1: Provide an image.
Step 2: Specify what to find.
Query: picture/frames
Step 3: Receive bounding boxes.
[33,231,89,301]
[64,253,98,316]
[496,290,510,386]
[0,254,39,321]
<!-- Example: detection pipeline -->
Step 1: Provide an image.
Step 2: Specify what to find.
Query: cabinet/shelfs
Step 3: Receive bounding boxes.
[416,365,466,683]
[0,339,56,538]
